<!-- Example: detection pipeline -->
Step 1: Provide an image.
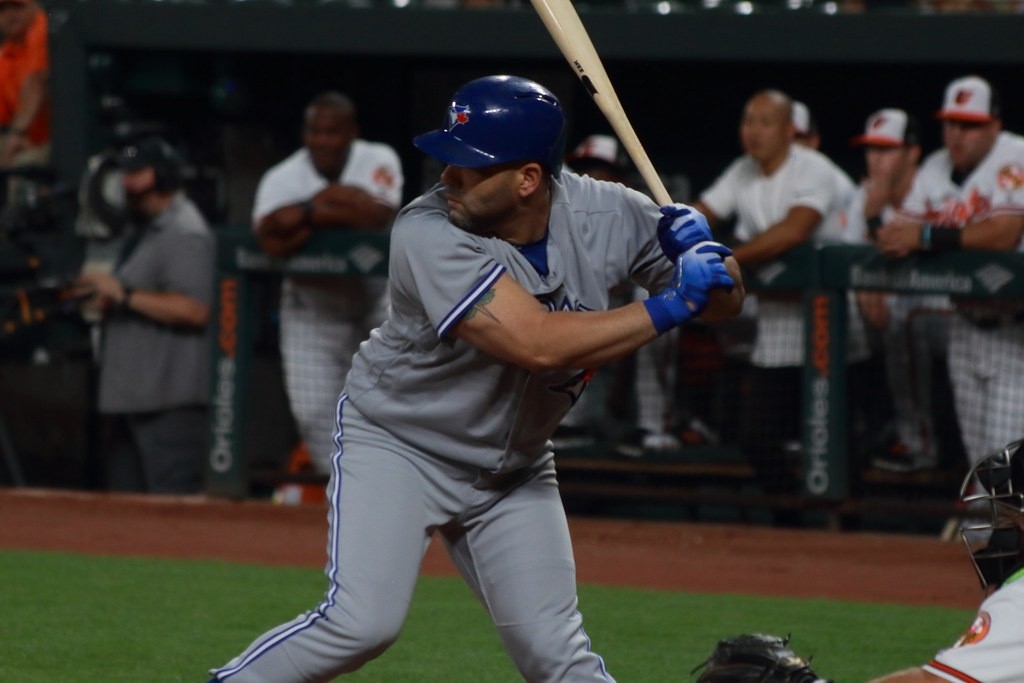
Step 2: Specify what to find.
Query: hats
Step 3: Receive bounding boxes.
[106,137,186,179]
[960,439,1024,588]
[936,75,1002,124]
[789,101,821,136]
[855,107,924,148]
[576,133,629,167]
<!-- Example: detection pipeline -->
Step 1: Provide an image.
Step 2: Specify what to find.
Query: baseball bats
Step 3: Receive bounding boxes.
[530,0,675,207]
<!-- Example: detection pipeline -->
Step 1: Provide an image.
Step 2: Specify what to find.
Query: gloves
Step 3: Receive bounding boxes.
[656,202,711,267]
[642,242,735,336]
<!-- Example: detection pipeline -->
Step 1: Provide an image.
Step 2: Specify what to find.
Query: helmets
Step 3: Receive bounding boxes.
[412,75,567,168]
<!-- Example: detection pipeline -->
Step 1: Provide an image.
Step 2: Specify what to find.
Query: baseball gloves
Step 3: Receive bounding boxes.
[696,632,835,683]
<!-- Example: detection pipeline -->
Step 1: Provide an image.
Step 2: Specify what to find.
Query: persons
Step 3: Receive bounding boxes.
[75,137,217,494]
[207,75,734,681]
[252,95,405,475]
[876,76,1024,546]
[841,104,967,472]
[791,100,824,150]
[538,125,682,452]
[686,91,870,456]
[693,441,1024,682]
[0,0,48,209]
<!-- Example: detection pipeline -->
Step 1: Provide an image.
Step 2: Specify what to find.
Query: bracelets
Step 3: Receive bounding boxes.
[4,124,27,136]
[119,286,134,306]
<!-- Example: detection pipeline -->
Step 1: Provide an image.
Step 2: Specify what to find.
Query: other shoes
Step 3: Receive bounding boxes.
[541,423,604,450]
[616,427,649,456]
[691,414,749,452]
[873,422,939,473]
[642,431,687,455]
